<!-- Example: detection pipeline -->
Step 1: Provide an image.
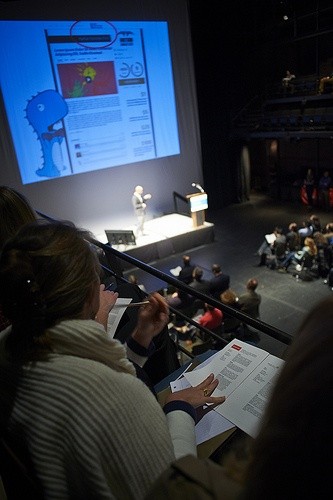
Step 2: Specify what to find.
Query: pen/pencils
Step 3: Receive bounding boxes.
[112,300,151,308]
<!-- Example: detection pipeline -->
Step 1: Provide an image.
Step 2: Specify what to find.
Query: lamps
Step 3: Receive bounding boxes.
[105,230,136,245]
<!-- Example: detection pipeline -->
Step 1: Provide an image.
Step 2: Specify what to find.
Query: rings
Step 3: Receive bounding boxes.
[201,387,208,397]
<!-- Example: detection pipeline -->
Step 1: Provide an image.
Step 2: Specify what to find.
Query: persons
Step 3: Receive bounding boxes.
[253,227,287,268]
[287,223,301,242]
[199,302,223,336]
[1,220,226,500]
[166,287,199,320]
[277,237,317,272]
[298,218,314,243]
[237,279,261,320]
[217,288,242,332]
[318,171,332,211]
[210,262,230,300]
[304,168,316,210]
[177,255,196,284]
[132,185,152,237]
[281,70,296,90]
[0,185,119,332]
[188,267,212,296]
[145,291,333,500]
[312,222,333,290]
[309,215,321,232]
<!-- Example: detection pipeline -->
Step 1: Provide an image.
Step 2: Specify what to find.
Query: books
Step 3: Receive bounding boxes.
[183,338,287,441]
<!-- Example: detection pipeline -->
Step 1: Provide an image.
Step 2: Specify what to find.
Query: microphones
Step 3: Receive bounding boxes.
[192,182,204,192]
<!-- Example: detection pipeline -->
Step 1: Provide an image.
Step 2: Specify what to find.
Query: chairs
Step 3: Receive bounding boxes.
[249,106,333,130]
[260,221,333,281]
[266,71,333,98]
[154,278,261,356]
